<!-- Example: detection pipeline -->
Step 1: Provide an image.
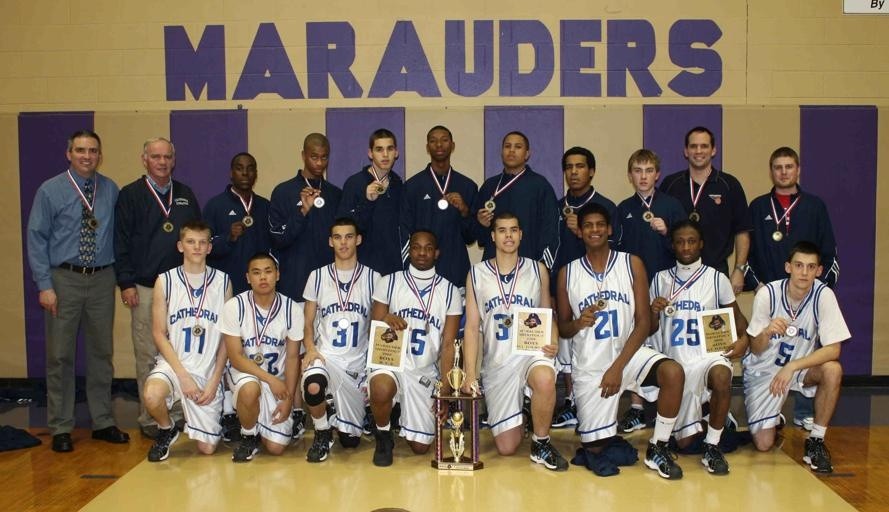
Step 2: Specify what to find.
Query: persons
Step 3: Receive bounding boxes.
[270,133,347,442]
[473,132,562,428]
[143,226,230,462]
[744,241,853,473]
[747,148,838,432]
[366,229,462,465]
[114,138,200,437]
[550,147,637,430]
[613,151,687,434]
[462,213,569,471]
[298,221,382,462]
[666,128,750,434]
[218,252,305,457]
[556,204,682,481]
[398,127,479,337]
[204,154,270,442]
[650,222,747,475]
[25,128,131,453]
[340,129,405,275]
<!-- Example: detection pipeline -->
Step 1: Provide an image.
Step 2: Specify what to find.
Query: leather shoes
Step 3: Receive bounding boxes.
[52,434,73,454]
[93,426,130,443]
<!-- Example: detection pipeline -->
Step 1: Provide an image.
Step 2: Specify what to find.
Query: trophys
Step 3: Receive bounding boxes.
[431,338,485,471]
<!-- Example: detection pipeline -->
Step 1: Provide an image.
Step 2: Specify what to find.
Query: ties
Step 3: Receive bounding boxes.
[79,179,97,268]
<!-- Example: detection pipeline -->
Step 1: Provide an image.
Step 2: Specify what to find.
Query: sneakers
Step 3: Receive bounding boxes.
[702,440,720,472]
[305,424,335,462]
[727,413,737,434]
[550,398,578,427]
[795,415,815,430]
[373,425,395,467]
[530,435,570,471]
[291,408,307,440]
[618,408,647,434]
[644,438,683,480]
[148,418,179,462]
[233,433,259,462]
[802,437,832,472]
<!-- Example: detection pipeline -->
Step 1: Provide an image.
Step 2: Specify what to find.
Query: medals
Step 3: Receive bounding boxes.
[246,290,277,365]
[405,267,437,333]
[369,164,394,195]
[330,263,359,330]
[767,188,802,242]
[559,187,596,224]
[581,248,613,310]
[177,262,211,335]
[229,185,255,229]
[483,168,528,211]
[64,169,103,230]
[780,279,814,338]
[685,168,711,226]
[663,263,704,315]
[490,258,521,329]
[425,164,453,211]
[142,173,177,232]
[632,189,656,222]
[299,174,326,208]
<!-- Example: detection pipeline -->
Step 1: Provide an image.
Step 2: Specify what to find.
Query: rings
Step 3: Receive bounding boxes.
[124,300,128,304]
